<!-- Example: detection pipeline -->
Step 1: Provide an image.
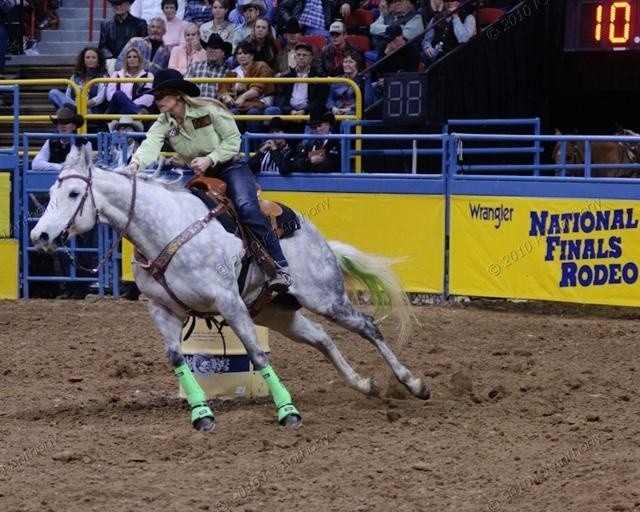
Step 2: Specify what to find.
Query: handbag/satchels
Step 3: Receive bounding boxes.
[35,15,52,30]
[233,97,267,111]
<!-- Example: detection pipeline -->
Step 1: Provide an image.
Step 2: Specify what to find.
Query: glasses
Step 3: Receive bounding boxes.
[154,92,174,100]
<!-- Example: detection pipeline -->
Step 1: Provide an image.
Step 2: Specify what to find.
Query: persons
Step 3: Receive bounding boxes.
[1,1,518,176]
[118,70,294,289]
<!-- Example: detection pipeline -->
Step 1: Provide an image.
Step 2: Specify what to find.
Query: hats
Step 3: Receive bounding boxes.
[329,22,346,34]
[136,69,200,97]
[108,0,135,4]
[304,98,335,125]
[295,42,313,51]
[235,0,268,18]
[282,18,306,33]
[200,33,232,55]
[383,25,402,41]
[49,108,84,127]
[112,118,144,132]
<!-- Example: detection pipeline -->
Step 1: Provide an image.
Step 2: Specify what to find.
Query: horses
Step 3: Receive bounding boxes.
[29,139,433,434]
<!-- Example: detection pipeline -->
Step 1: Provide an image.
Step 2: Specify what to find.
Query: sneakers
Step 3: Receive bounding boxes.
[269,272,295,289]
